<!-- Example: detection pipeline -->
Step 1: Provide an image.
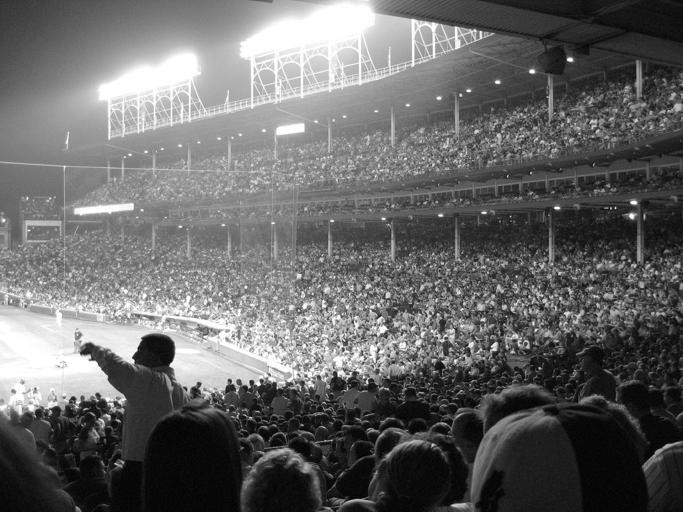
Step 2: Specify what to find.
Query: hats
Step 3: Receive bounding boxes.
[575,345,603,361]
[450,402,649,512]
